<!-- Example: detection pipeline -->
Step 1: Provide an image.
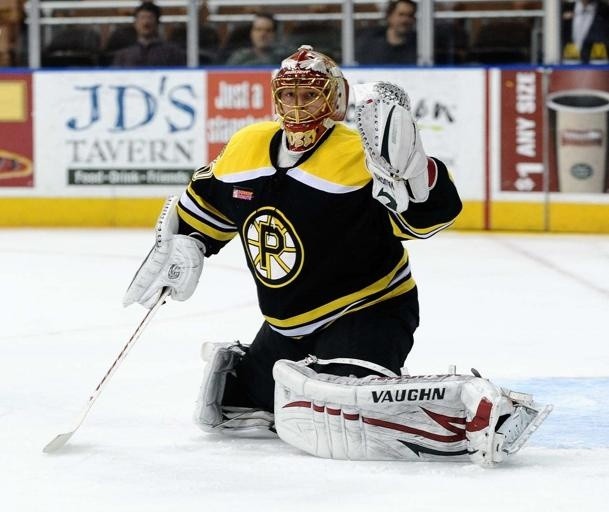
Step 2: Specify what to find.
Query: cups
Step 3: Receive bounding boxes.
[545,87,609,195]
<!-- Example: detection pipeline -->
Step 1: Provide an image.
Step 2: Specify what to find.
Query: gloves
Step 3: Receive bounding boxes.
[352,78,430,215]
[118,194,206,310]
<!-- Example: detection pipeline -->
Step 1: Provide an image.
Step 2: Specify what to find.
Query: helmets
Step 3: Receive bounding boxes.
[270,44,350,155]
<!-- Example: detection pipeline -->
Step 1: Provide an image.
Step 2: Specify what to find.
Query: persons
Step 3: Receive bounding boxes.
[223,10,290,66]
[120,44,534,468]
[109,0,177,69]
[352,0,417,65]
[562,0,607,66]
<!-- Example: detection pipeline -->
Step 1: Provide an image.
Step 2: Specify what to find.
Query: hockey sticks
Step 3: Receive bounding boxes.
[42,287,172,452]
